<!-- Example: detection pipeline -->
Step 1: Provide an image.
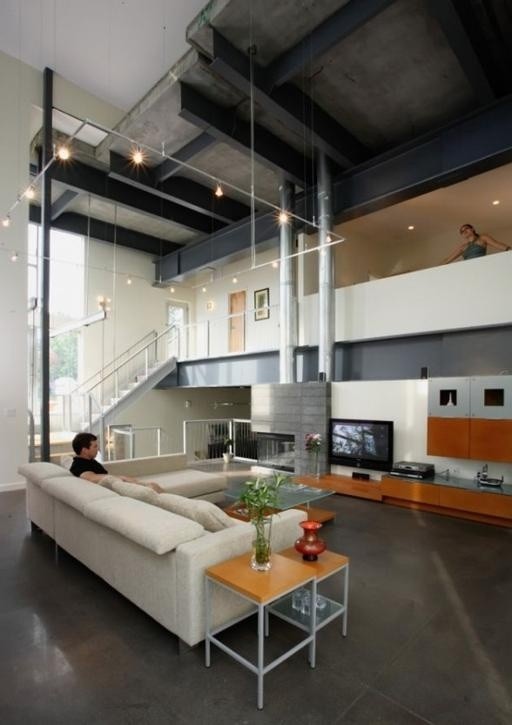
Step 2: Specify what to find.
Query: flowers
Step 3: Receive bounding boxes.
[304,431,324,474]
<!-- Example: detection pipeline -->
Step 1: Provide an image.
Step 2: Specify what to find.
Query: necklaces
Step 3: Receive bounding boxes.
[467,238,477,246]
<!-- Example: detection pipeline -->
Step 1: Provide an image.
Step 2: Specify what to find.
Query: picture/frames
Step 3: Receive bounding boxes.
[253,288,270,321]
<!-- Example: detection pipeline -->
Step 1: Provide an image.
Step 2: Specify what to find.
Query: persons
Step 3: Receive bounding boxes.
[69,432,163,493]
[443,223,512,265]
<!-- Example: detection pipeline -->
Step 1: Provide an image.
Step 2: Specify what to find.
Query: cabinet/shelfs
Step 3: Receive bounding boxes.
[381,473,511,529]
[426,375,512,463]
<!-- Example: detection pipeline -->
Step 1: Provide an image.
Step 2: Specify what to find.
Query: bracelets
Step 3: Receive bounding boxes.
[504,246,510,251]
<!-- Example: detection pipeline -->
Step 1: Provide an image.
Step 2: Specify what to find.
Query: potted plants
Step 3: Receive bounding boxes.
[222,435,235,465]
[232,469,293,572]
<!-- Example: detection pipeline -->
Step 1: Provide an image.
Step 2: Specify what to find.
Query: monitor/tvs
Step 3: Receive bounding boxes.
[329,417,393,472]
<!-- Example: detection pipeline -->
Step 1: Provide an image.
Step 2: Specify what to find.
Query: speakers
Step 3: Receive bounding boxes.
[318,372,326,383]
[421,367,429,378]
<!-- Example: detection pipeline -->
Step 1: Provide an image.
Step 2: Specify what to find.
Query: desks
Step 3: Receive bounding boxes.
[291,473,383,502]
[204,546,349,712]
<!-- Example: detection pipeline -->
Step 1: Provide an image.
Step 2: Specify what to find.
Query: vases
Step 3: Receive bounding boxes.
[294,521,327,562]
[312,452,321,476]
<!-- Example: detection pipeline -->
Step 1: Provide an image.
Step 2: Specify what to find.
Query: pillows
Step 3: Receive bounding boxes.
[98,474,235,532]
